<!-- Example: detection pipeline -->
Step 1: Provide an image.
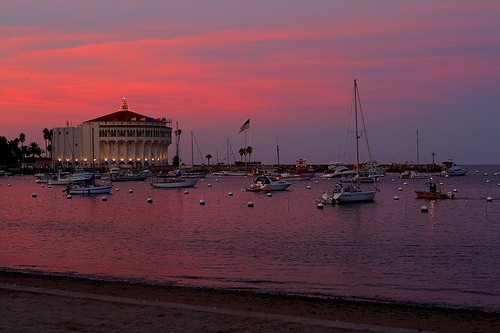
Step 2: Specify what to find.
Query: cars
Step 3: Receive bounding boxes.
[24,161,75,172]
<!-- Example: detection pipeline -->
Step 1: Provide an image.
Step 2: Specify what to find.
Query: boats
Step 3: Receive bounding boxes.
[400,129,469,179]
[35,127,151,194]
[211,136,314,191]
[320,160,385,183]
[414,179,454,199]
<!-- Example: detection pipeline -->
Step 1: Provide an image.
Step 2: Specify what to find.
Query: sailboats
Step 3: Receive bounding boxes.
[321,79,383,204]
[149,121,208,189]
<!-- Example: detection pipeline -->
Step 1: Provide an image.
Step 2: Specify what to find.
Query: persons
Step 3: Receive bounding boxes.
[430,183,437,196]
[322,180,343,201]
[173,171,181,183]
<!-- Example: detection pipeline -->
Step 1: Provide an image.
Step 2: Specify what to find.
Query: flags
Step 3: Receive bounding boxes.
[239,117,250,133]
